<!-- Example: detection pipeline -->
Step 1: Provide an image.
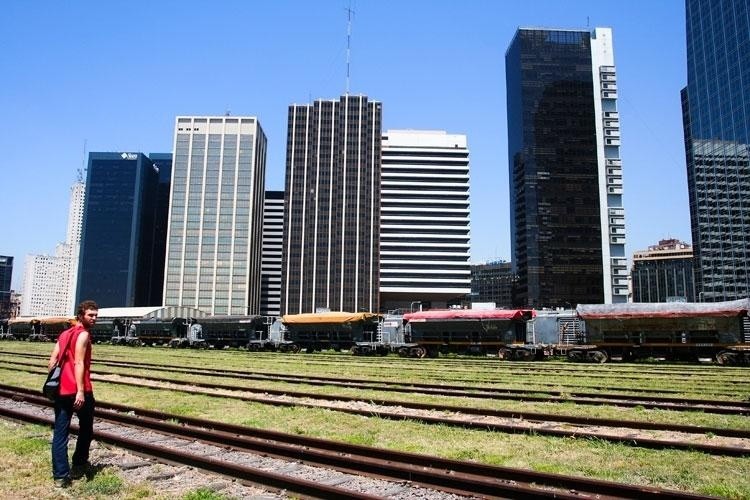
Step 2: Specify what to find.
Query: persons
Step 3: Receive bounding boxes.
[48,300,102,488]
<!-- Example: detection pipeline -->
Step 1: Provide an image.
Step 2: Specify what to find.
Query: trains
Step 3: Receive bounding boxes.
[0,295,750,366]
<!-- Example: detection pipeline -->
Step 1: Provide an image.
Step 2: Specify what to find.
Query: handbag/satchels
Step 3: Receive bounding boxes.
[43,365,61,401]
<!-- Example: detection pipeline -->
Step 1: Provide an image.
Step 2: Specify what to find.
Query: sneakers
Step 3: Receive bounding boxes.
[54,478,65,488]
